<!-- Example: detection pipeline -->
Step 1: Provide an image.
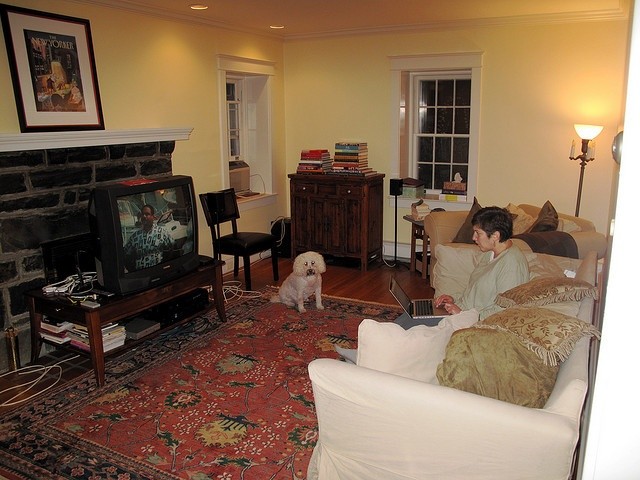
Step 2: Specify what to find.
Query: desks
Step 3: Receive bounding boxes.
[404,215,429,278]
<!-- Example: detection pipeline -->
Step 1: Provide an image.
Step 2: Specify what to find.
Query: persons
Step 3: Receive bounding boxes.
[62,50,75,83]
[70,87,80,101]
[123,206,173,269]
[334,207,530,365]
[43,77,55,92]
[50,94,66,112]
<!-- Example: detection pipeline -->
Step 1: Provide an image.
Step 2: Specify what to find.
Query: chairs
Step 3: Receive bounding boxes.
[200,188,279,290]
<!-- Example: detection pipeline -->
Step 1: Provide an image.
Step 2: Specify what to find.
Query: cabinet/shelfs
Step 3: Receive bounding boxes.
[30,254,227,387]
[289,173,386,270]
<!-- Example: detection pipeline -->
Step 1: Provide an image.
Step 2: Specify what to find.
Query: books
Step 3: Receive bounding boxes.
[331,142,377,176]
[411,198,429,222]
[72,322,127,353]
[39,320,69,344]
[438,191,466,202]
[297,149,332,174]
[401,177,424,197]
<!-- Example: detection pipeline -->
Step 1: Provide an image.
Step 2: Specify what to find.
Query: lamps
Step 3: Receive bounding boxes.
[567,122,603,163]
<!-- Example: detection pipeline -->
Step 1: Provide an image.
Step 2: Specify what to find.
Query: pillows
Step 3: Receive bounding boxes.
[529,201,558,235]
[357,308,477,384]
[473,308,603,366]
[504,202,534,238]
[494,276,599,312]
[440,330,560,410]
[450,195,483,245]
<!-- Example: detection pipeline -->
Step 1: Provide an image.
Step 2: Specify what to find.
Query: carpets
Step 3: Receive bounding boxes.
[0,287,400,480]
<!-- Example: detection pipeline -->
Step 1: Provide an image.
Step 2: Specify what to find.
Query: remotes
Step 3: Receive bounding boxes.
[81,300,102,311]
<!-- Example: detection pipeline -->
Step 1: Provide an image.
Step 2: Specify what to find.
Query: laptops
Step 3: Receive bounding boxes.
[389,275,452,319]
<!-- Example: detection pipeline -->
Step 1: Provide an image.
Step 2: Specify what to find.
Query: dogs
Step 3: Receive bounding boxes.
[270,251,327,314]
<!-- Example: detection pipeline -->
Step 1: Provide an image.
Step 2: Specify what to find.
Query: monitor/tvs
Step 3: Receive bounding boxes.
[88,171,200,295]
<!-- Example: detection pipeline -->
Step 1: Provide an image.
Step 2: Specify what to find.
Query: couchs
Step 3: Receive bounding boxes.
[423,202,611,289]
[306,246,599,467]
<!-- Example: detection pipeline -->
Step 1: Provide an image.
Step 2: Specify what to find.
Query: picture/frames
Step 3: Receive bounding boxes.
[1,4,105,132]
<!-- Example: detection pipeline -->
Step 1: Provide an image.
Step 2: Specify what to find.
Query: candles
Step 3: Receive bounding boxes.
[587,141,593,160]
[592,141,597,160]
[569,138,575,159]
